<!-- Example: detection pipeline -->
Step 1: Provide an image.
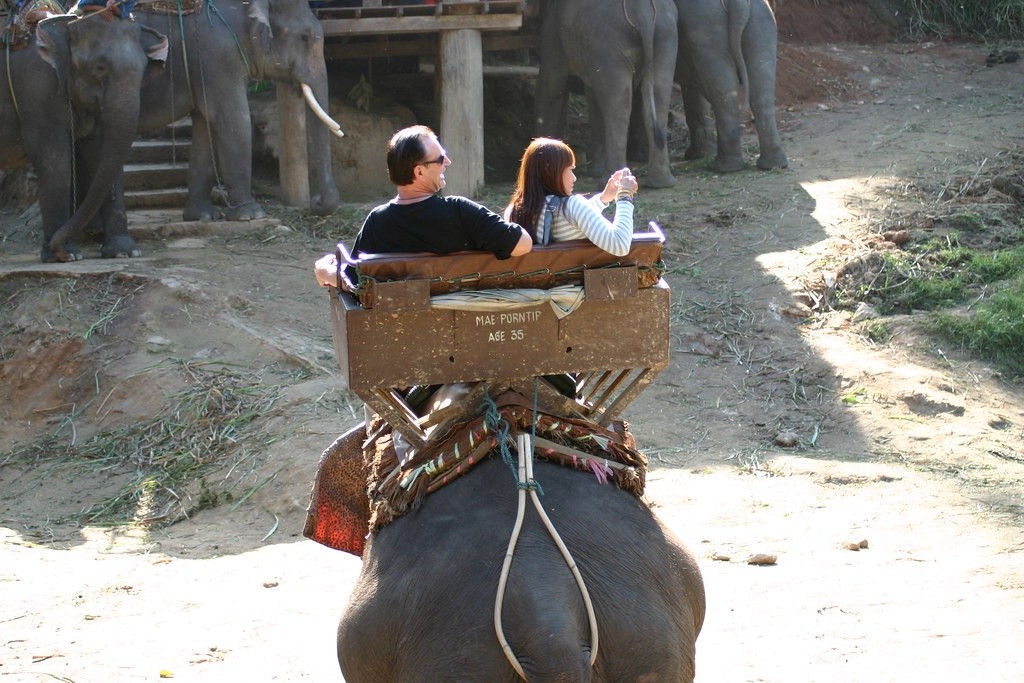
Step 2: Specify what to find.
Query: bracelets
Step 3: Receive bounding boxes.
[616,189,634,204]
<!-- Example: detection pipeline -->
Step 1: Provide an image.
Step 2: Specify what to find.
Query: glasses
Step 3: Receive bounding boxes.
[418,155,444,166]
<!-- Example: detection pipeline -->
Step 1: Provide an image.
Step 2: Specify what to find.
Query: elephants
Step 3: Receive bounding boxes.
[521,1,787,190]
[0,0,346,264]
[302,370,707,683]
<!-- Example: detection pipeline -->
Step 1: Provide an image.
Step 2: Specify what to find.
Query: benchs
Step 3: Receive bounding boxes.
[328,221,673,391]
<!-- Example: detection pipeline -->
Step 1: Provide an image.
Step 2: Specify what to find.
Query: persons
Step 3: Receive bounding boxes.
[504,137,638,406]
[315,125,532,399]
[25,0,137,23]
